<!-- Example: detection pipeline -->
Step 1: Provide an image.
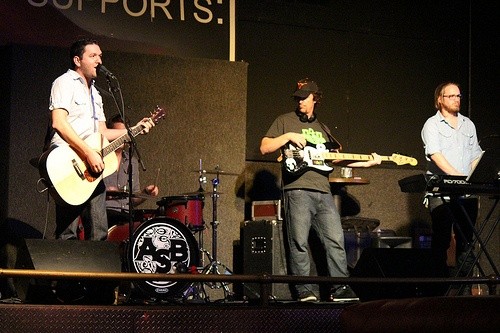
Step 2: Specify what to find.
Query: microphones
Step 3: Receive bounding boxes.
[97,64,116,80]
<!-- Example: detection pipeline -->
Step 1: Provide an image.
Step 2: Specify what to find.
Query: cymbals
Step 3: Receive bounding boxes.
[206,170,240,176]
[185,191,223,196]
[105,188,154,201]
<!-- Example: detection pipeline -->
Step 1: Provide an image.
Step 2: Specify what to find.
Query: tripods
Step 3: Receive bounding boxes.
[174,181,262,302]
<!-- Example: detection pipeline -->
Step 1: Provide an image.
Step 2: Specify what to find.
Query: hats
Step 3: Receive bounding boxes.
[291,78,319,99]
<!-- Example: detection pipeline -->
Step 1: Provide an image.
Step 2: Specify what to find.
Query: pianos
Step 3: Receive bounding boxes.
[397,174,500,295]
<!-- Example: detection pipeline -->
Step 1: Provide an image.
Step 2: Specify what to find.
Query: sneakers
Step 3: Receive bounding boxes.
[332,287,360,301]
[296,292,317,302]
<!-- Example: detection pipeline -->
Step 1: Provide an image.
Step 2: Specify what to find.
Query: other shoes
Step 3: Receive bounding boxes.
[145,297,155,303]
[462,289,472,297]
[161,299,177,305]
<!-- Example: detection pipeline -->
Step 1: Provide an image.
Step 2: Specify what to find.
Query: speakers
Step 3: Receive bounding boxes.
[354,248,433,299]
[15,240,122,305]
[239,220,320,300]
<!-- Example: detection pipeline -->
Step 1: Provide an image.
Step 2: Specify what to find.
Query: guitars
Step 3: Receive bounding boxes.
[39,105,166,209]
[281,141,418,177]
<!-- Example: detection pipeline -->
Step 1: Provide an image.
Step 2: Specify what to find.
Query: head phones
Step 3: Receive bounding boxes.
[295,107,317,123]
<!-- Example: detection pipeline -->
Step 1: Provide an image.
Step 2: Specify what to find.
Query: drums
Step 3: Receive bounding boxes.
[162,196,205,230]
[106,214,201,301]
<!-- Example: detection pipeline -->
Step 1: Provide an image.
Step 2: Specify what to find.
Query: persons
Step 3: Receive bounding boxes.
[421,81,483,296]
[49,37,155,243]
[260,77,359,301]
[104,113,159,211]
